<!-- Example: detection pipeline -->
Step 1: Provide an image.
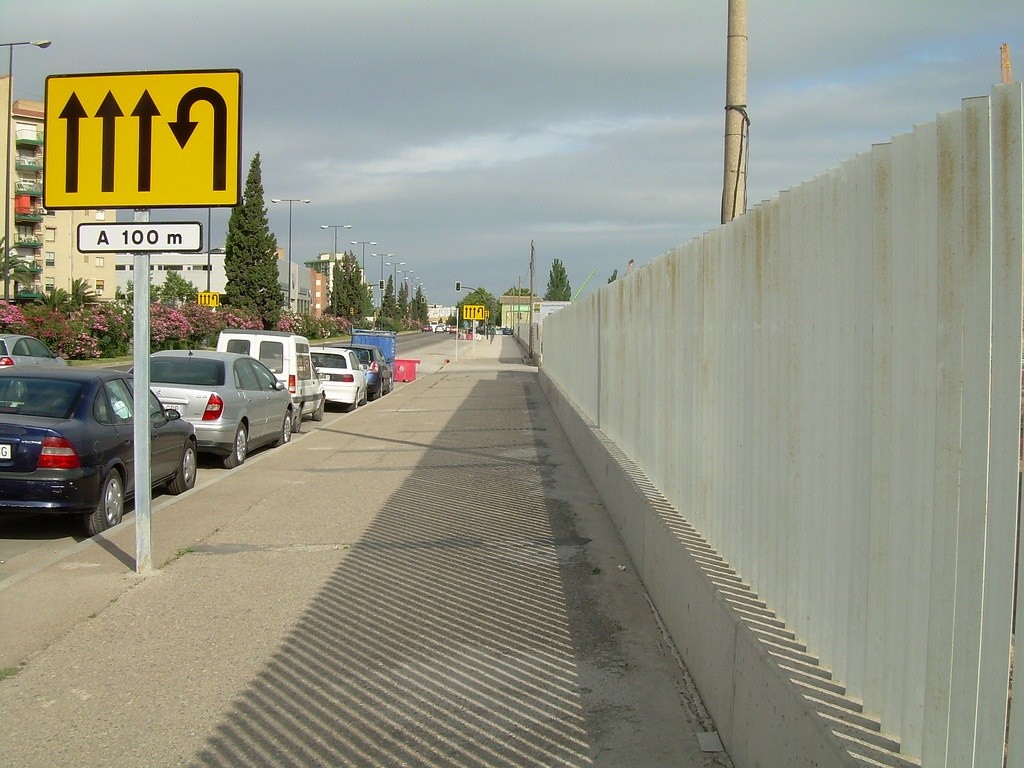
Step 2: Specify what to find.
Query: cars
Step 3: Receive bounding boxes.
[108,350,296,469]
[297,347,367,412]
[450,326,457,333]
[0,365,198,537]
[422,325,433,332]
[502,328,513,335]
[446,327,451,332]
[0,334,67,368]
[435,326,444,333]
[333,345,393,401]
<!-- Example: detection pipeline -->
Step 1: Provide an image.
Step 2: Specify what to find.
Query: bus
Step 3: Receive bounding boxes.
[430,321,438,333]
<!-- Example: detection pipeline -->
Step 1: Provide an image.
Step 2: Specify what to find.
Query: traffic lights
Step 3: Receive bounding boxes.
[456,282,460,291]
[379,280,384,290]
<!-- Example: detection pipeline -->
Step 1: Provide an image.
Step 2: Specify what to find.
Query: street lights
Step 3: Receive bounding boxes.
[372,253,394,330]
[386,262,406,299]
[0,41,52,305]
[321,225,352,318]
[397,271,425,300]
[271,199,311,310]
[351,241,378,284]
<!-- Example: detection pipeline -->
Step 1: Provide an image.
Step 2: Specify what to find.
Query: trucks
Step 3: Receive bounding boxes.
[216,329,326,432]
